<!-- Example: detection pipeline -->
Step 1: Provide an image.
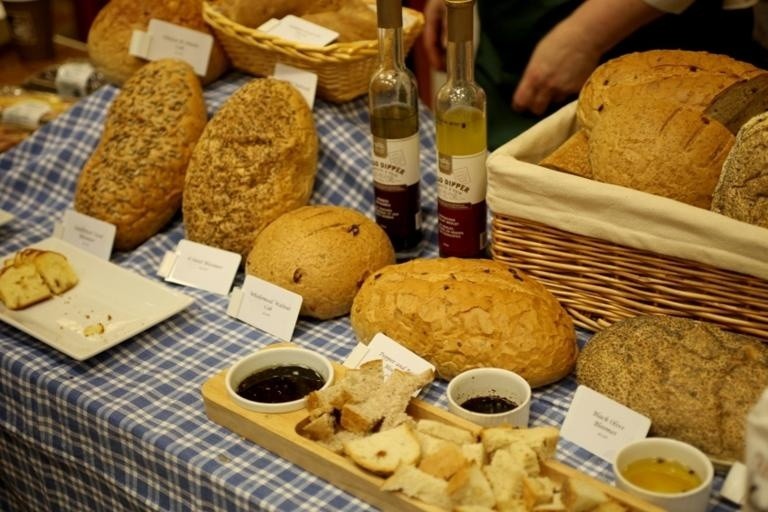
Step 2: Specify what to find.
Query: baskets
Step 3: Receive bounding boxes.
[202,2,425,103]
[485,100,763,341]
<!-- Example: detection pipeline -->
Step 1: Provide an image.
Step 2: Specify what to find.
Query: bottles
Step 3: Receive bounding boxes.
[365,0,425,262]
[431,0,489,260]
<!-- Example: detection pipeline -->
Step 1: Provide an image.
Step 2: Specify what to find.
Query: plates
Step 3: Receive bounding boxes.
[0,234,196,362]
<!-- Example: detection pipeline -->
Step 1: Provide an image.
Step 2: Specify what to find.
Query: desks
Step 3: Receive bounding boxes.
[0,38,751,511]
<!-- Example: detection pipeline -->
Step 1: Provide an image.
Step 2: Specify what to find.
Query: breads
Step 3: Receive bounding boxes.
[1,247,78,311]
[576,304,768,467]
[297,357,608,512]
[347,250,579,392]
[73,57,207,253]
[538,49,768,230]
[182,76,318,271]
[87,0,229,91]
[295,8,380,50]
[242,203,397,320]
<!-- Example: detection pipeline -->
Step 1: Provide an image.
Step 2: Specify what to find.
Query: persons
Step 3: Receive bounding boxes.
[512,0,767,115]
[402,0,582,106]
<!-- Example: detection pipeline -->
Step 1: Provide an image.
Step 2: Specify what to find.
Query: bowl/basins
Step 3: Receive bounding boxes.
[611,436,714,511]
[445,365,531,434]
[226,345,332,413]
